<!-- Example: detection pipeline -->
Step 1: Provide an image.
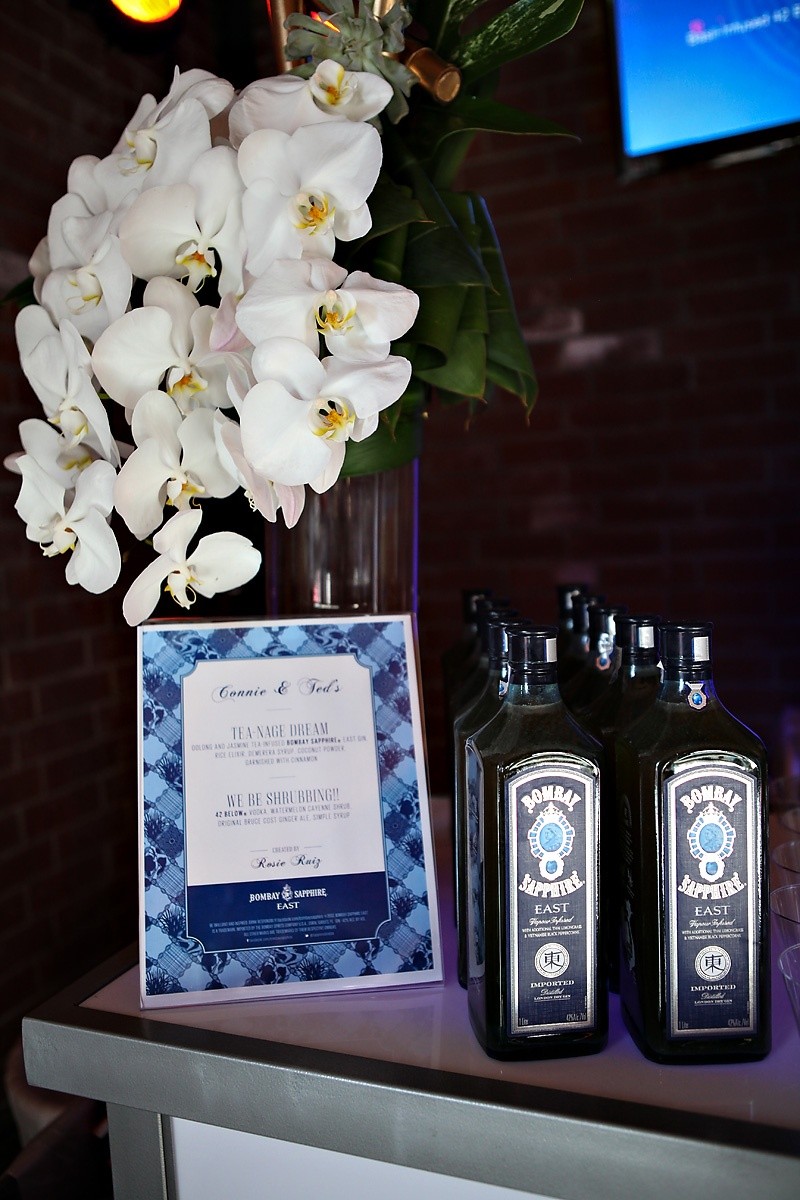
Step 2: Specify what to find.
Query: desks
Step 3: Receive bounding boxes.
[18,785,800,1199]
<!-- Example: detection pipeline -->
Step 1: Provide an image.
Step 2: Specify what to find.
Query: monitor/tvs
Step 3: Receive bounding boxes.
[601,0,800,179]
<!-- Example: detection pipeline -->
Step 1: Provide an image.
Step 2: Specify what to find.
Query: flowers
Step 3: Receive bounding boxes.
[9,0,602,627]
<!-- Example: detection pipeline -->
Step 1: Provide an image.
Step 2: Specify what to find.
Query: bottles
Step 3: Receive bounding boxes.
[452,588,777,1066]
